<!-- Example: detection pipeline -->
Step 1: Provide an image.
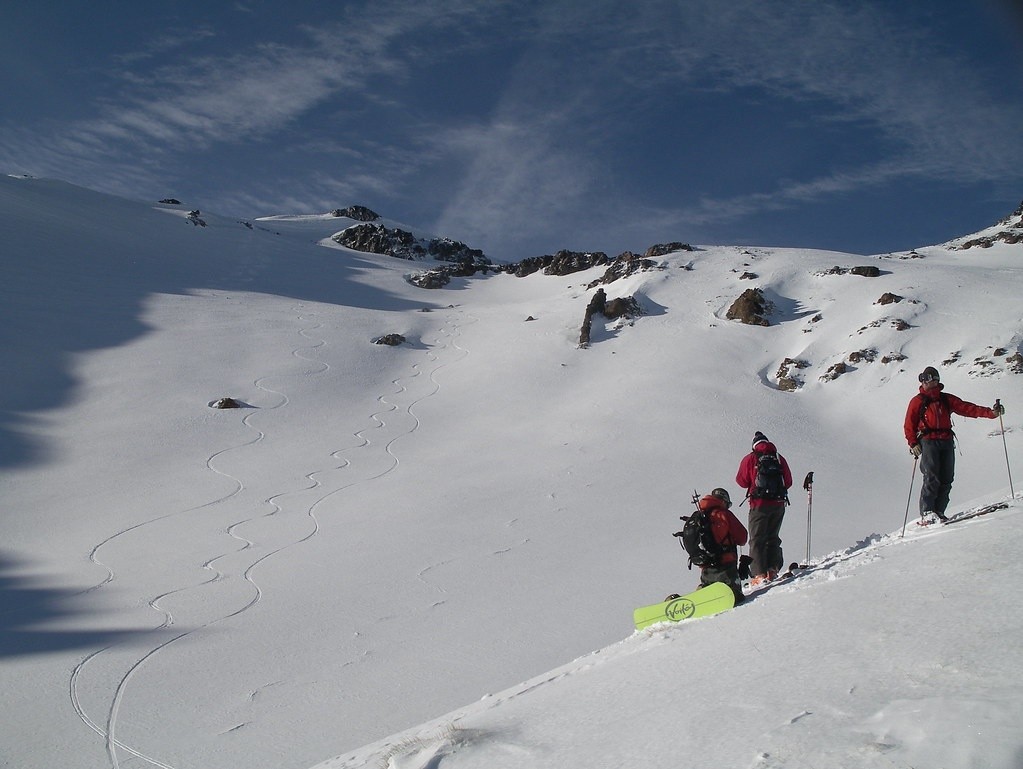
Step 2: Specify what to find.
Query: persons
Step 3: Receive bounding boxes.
[904,366,1005,526]
[735,430,792,587]
[665,487,748,606]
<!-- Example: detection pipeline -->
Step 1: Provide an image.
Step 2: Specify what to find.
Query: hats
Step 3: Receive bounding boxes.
[922,367,940,382]
[752,431,769,450]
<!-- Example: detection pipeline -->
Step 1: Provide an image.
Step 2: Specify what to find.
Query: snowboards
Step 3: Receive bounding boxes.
[631,580,738,632]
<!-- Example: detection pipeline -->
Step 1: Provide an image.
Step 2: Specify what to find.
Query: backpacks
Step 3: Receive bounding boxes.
[672,505,733,569]
[748,450,790,505]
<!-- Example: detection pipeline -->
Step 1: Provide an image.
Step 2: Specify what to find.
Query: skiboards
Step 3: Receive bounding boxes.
[744,572,792,592]
[907,500,1010,533]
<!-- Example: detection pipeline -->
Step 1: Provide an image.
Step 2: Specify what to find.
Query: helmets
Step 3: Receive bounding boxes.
[712,488,732,508]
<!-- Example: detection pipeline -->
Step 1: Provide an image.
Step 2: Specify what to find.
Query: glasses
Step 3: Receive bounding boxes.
[918,373,933,382]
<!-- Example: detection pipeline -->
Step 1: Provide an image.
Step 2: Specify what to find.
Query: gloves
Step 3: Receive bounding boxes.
[913,444,922,456]
[993,404,1005,417]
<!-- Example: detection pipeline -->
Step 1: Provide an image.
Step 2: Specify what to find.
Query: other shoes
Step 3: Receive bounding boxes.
[935,512,948,523]
[751,569,778,586]
[922,510,935,524]
